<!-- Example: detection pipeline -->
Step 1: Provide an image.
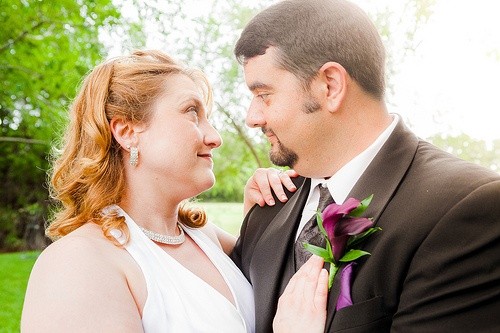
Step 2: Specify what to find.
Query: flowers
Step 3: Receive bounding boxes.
[302,192,383,292]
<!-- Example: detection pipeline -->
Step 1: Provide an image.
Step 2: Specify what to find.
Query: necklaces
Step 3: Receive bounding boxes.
[134,224,188,245]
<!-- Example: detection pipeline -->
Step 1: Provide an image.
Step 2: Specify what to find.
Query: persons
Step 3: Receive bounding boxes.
[18,49,331,333]
[228,0,500,333]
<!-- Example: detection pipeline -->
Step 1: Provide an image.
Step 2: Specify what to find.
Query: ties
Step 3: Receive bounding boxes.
[296,183,336,275]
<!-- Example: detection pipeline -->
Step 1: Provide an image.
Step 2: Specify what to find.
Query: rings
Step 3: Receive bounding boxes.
[279,168,286,175]
